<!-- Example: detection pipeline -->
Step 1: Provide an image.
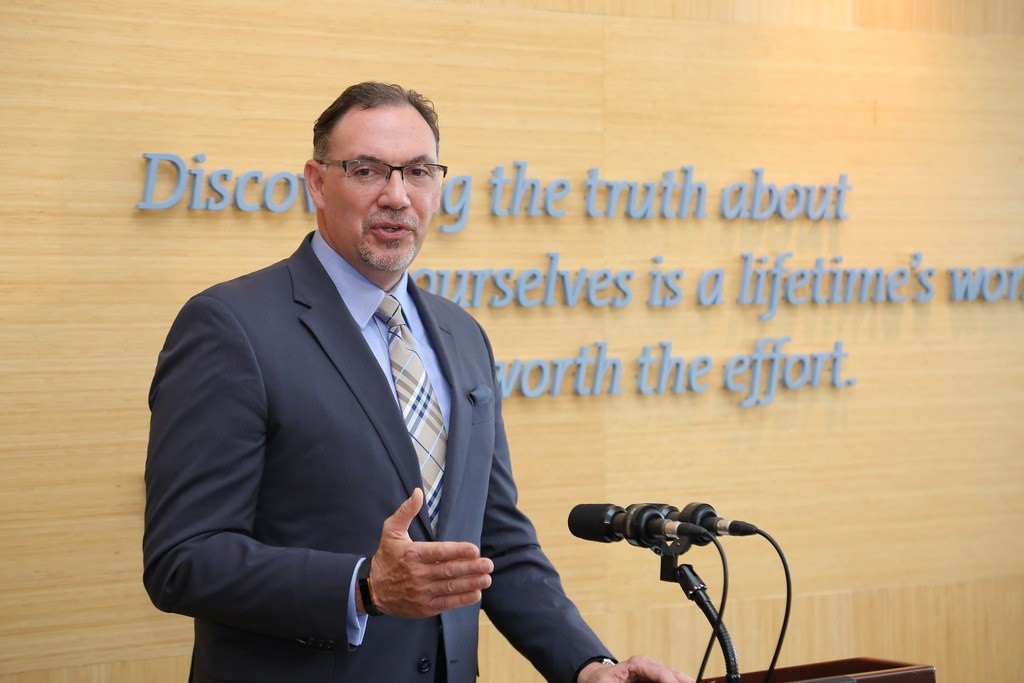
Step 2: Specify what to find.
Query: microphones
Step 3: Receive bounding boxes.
[625,504,759,546]
[569,503,706,543]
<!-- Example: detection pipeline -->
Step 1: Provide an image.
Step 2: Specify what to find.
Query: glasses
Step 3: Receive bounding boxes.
[313,158,448,193]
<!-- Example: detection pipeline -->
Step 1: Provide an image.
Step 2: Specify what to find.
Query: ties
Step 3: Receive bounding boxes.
[374,293,448,539]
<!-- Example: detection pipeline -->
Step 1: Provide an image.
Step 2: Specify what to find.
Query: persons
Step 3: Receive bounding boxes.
[142,81,702,680]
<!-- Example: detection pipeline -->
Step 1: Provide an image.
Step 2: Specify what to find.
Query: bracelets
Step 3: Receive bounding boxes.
[357,554,383,617]
[572,657,617,683]
